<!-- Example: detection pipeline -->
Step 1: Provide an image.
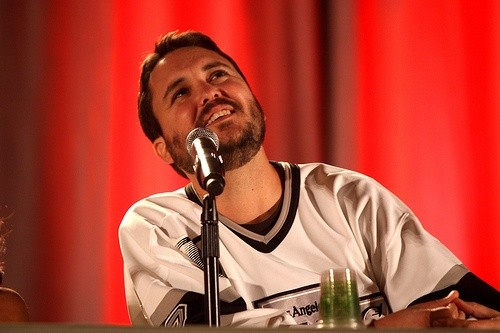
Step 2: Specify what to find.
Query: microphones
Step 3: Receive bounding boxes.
[186,127,225,196]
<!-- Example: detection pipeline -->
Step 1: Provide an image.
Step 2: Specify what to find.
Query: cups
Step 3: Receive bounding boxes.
[318,268,366,329]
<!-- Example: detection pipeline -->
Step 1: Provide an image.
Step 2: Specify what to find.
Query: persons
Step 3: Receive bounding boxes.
[119,29,500,333]
